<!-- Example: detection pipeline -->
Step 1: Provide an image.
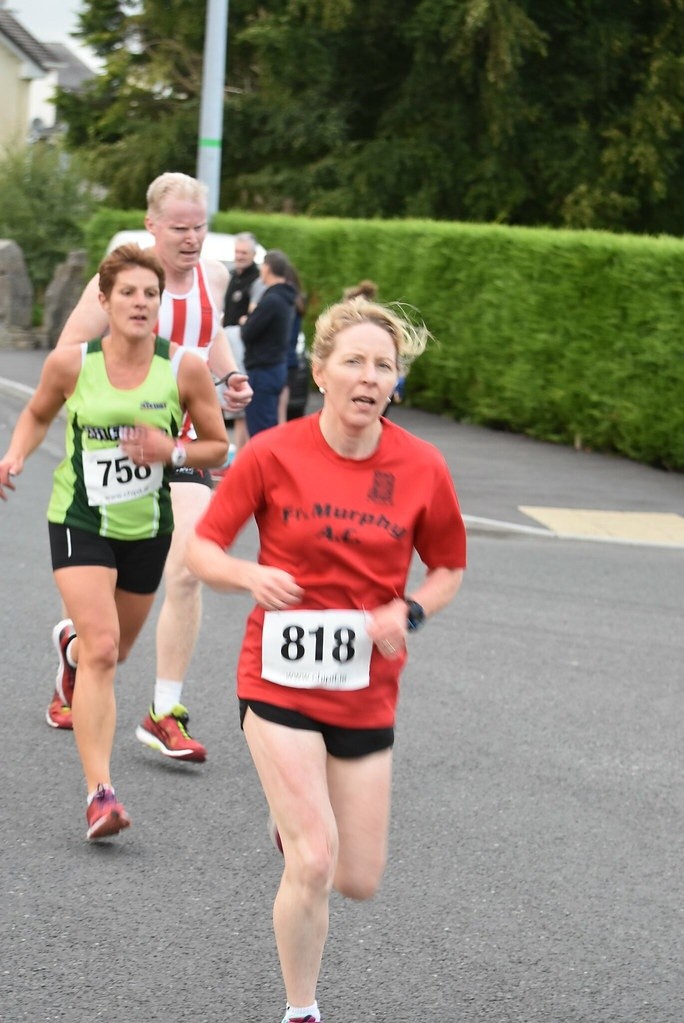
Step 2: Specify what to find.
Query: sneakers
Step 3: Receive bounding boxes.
[135,699,207,763]
[46,690,74,730]
[210,465,232,481]
[51,618,78,710]
[85,790,130,842]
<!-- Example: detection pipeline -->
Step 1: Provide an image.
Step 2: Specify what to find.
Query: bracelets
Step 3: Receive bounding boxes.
[215,371,242,386]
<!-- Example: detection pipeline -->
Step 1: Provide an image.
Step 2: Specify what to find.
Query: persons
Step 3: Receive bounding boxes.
[222,231,262,329]
[182,281,467,1022]
[45,173,253,765]
[2,244,232,840]
[238,251,309,439]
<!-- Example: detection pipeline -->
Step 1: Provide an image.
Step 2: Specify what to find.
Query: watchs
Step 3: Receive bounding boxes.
[171,436,187,470]
[404,599,426,633]
